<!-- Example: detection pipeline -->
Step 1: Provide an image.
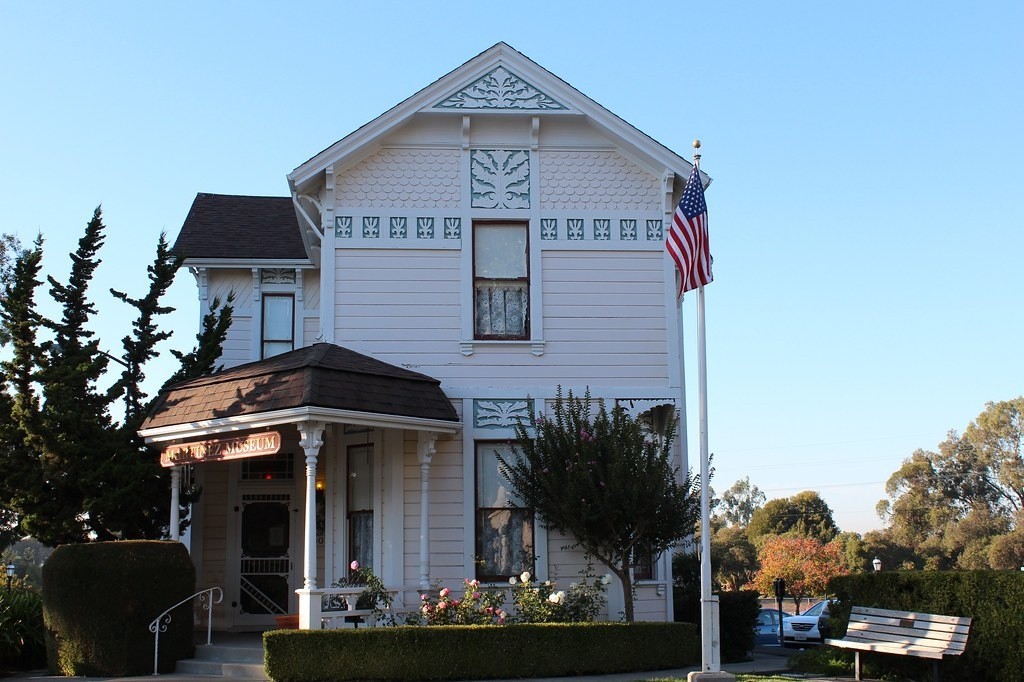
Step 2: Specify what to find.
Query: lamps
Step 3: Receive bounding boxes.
[5,561,15,580]
[316,480,324,504]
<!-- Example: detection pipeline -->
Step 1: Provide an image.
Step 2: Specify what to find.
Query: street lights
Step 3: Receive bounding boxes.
[4,560,16,594]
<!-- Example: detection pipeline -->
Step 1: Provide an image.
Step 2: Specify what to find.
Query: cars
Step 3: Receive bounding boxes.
[754,608,793,646]
[777,597,840,649]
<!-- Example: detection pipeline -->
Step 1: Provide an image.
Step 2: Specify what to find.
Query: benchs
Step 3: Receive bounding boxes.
[824,606,972,682]
[273,615,299,629]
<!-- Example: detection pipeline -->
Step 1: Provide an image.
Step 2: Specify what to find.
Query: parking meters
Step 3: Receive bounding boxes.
[772,577,786,646]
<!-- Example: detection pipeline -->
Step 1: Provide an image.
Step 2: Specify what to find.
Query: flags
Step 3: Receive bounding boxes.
[666,154,713,302]
